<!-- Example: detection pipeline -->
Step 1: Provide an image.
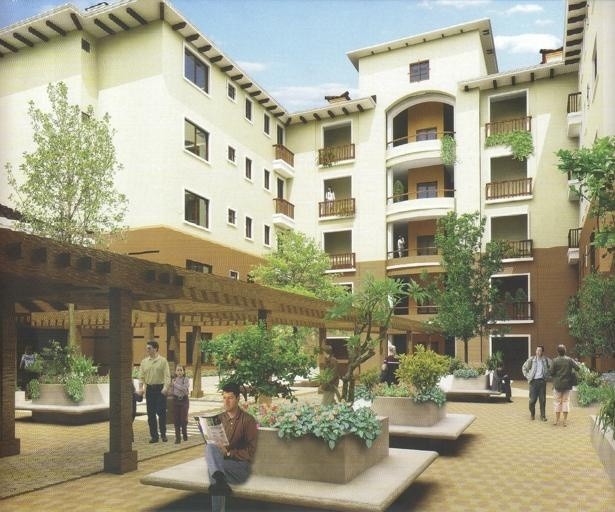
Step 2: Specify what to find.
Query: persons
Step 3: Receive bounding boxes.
[204,383,258,512]
[492,362,513,402]
[170,364,190,444]
[398,235,404,257]
[130,383,144,444]
[138,341,171,443]
[549,345,580,427]
[521,345,552,422]
[380,345,400,388]
[326,186,336,214]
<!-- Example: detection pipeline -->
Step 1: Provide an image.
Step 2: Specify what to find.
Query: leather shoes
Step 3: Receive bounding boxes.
[208,484,233,496]
[183,435,187,441]
[162,437,168,442]
[149,437,159,443]
[175,438,181,444]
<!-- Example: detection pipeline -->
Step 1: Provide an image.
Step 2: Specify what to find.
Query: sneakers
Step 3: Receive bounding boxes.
[529,415,568,428]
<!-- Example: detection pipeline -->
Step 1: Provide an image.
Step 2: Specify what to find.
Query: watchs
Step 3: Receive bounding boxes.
[224,451,231,459]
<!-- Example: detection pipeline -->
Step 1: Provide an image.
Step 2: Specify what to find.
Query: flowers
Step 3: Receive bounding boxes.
[239,394,382,451]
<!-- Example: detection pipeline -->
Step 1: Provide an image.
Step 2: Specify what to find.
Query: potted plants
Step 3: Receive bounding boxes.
[504,291,515,320]
[515,288,528,320]
[14,341,111,425]
[141,320,440,511]
[350,341,476,442]
[439,351,505,400]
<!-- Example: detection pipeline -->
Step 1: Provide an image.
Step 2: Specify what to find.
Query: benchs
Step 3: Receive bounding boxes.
[140,445,440,510]
[351,399,476,447]
[14,397,111,417]
[441,378,513,395]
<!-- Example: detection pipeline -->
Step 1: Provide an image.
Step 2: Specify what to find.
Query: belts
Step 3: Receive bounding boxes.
[174,394,188,400]
